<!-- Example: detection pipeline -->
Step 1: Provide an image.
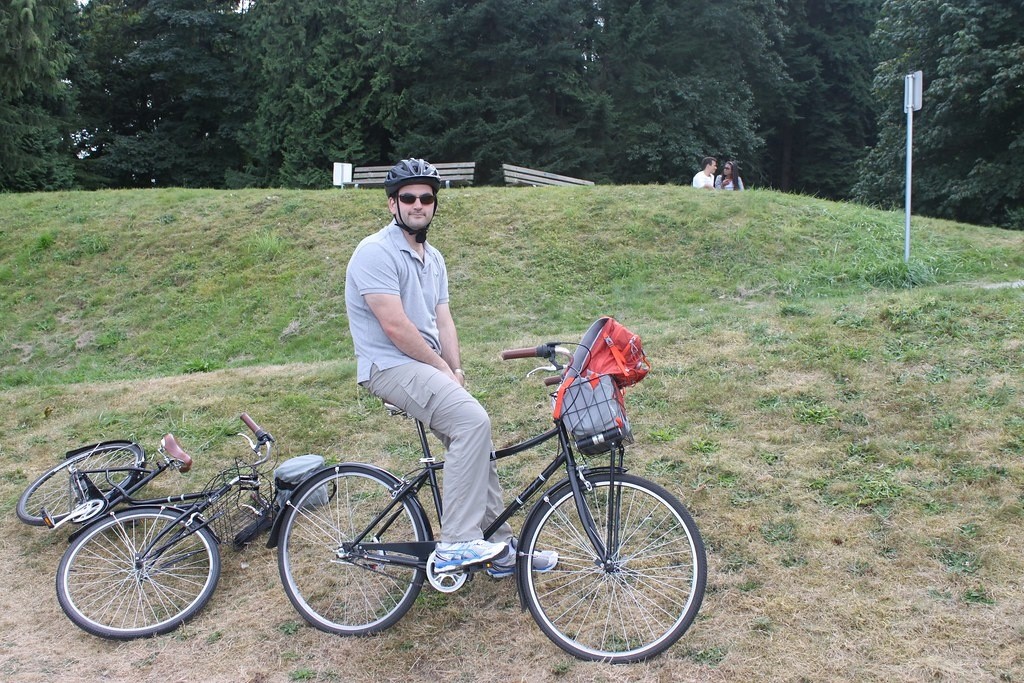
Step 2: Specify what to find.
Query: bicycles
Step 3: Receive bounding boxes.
[266,341,709,664]
[16,411,277,641]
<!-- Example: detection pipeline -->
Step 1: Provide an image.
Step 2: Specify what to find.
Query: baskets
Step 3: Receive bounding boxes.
[202,456,275,551]
[549,374,635,459]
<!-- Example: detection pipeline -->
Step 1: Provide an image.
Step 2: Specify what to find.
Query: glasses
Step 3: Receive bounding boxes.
[724,167,732,170]
[394,192,435,204]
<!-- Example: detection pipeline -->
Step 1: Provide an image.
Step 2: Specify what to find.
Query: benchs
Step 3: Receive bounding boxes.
[343,161,475,188]
[503,164,595,187]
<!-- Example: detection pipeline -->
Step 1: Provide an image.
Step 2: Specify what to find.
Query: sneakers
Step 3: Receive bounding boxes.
[486,536,559,578]
[434,539,510,573]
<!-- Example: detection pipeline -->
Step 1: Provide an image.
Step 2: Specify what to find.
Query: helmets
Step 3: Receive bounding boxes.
[384,157,441,198]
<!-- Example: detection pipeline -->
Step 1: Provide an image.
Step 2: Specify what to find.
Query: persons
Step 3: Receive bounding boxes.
[693,157,717,188]
[715,160,744,191]
[344,158,559,579]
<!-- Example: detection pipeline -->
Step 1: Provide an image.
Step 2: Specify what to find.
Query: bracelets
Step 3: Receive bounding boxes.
[454,369,464,376]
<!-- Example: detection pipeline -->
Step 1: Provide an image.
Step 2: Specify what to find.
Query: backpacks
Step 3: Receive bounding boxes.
[553,315,651,442]
